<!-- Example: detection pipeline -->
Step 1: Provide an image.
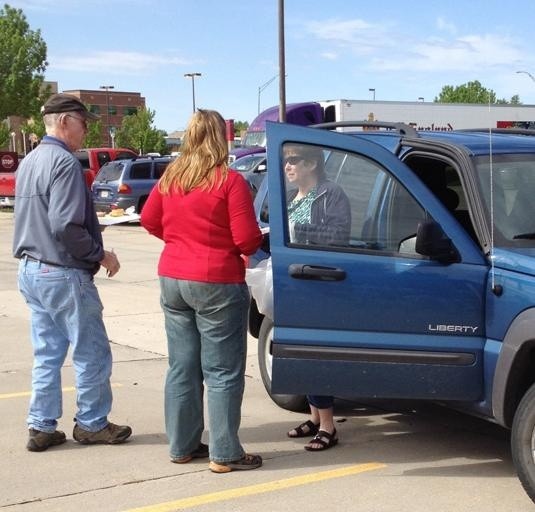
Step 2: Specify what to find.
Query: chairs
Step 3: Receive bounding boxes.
[506,180,535,238]
[428,184,482,251]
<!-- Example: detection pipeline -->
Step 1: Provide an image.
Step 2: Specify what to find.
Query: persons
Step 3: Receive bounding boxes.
[260,143,351,452]
[139,109,265,472]
[12,93,132,451]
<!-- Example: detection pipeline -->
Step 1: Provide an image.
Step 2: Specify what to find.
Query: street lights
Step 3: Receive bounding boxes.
[21,128,27,158]
[258,74,288,114]
[99,85,115,148]
[184,74,202,113]
[418,98,424,102]
[369,89,375,101]
[516,70,534,82]
[11,131,16,153]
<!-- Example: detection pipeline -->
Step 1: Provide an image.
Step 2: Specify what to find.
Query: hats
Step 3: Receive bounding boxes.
[40,92,102,123]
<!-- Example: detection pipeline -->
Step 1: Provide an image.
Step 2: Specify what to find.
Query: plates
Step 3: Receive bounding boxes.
[92,211,142,226]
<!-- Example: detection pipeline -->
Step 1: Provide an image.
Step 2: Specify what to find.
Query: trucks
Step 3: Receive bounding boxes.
[227,97,535,163]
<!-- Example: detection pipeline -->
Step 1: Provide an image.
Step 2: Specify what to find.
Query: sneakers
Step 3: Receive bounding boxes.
[73,418,133,447]
[28,424,66,452]
[167,443,209,463]
[208,451,262,473]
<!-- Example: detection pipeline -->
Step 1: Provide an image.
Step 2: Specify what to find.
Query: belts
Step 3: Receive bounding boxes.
[21,255,66,269]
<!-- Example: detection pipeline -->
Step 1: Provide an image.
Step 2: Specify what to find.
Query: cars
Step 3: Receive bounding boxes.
[228,152,267,196]
[0,154,28,208]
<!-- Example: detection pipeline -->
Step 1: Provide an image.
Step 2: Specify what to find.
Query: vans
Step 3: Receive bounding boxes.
[72,147,141,192]
[89,157,179,215]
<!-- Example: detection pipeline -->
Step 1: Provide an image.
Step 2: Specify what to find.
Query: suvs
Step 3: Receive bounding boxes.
[242,118,535,503]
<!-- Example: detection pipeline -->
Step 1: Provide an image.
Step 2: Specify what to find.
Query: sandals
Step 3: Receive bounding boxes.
[288,420,320,439]
[304,428,338,454]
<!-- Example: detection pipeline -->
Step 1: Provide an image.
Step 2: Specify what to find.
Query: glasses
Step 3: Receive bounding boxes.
[58,112,89,126]
[282,156,316,165]
